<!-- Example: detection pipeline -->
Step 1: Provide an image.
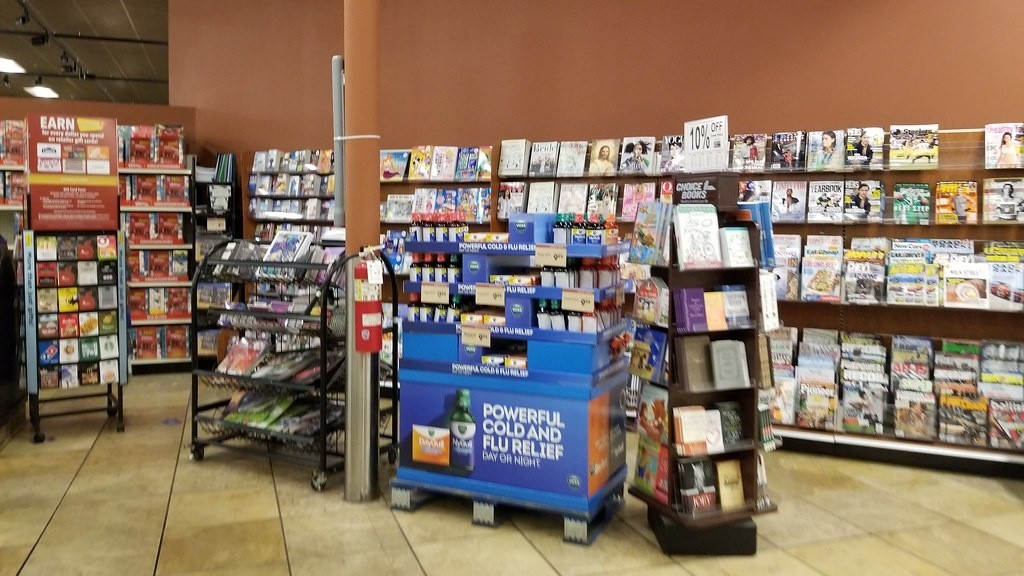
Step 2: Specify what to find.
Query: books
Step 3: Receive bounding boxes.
[0,0,1024,576]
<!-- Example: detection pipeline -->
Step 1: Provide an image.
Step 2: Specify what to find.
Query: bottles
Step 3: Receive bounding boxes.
[540,256,623,293]
[409,252,463,284]
[406,210,468,243]
[449,389,476,473]
[717,401,743,444]
[408,292,475,324]
[536,296,623,335]
[554,212,620,247]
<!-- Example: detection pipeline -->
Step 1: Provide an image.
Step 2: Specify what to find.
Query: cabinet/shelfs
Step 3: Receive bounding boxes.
[629,174,778,558]
[190,237,397,493]
[189,151,243,369]
[251,132,1016,470]
[389,240,629,546]
[0,124,196,371]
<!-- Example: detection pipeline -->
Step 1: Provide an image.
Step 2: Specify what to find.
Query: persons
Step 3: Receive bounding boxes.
[619,140,653,175]
[848,184,872,225]
[589,146,614,174]
[909,139,934,164]
[780,188,800,215]
[992,183,1024,222]
[810,130,843,171]
[992,131,1019,168]
[845,129,874,170]
[950,187,975,225]
[816,193,836,222]
[782,149,794,169]
[630,184,648,217]
[738,135,760,170]
[496,187,515,219]
[660,136,685,173]
